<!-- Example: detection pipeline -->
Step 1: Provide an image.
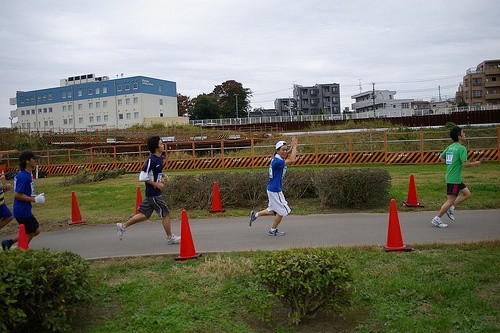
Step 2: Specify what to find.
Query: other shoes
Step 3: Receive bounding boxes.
[1,239,10,252]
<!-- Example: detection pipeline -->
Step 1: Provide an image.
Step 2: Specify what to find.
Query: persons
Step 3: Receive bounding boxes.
[248,136,298,236]
[430,128,481,227]
[0,161,13,227]
[2,151,45,250]
[116,136,181,244]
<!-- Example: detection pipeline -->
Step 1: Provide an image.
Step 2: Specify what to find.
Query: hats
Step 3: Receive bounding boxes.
[275,141,286,149]
[20,150,41,159]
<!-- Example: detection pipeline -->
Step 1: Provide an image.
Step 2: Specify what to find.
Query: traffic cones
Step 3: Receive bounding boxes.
[403,174,425,208]
[135,186,144,215]
[17,224,29,250]
[382,198,414,253]
[173,210,203,260]
[209,182,226,214]
[68,190,86,226]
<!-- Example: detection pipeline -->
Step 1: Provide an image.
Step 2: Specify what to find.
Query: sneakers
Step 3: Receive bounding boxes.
[431,218,448,228]
[446,208,456,221]
[249,210,257,226]
[268,229,285,236]
[116,223,124,240]
[167,234,181,244]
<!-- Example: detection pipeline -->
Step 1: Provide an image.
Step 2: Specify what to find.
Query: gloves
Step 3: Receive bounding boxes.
[35,193,45,204]
